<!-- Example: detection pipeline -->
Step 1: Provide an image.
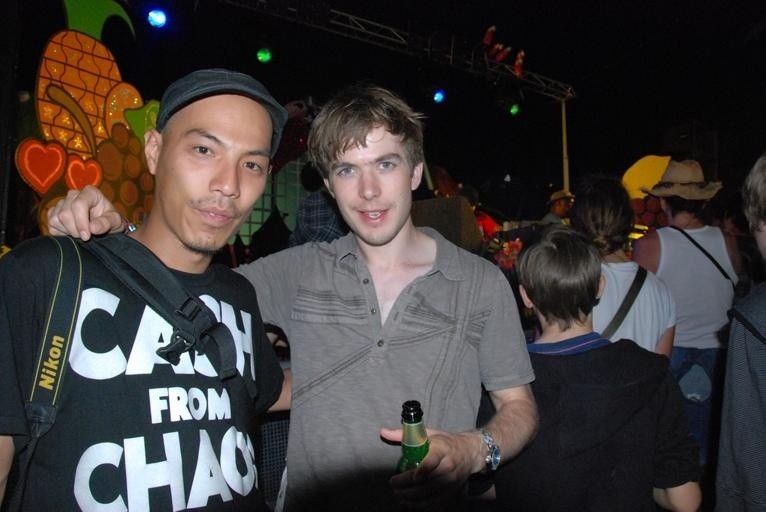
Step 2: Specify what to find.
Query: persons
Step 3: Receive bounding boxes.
[457,154,765,512]
[0,69,294,512]
[285,161,349,249]
[43,76,541,512]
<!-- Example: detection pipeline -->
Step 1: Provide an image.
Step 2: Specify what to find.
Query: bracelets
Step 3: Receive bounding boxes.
[121,215,131,235]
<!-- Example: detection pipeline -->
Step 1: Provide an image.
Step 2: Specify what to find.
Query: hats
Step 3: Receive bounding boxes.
[155,69,287,159]
[547,191,576,203]
[641,161,723,199]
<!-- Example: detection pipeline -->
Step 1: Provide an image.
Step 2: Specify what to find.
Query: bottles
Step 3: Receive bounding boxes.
[397,400,430,474]
